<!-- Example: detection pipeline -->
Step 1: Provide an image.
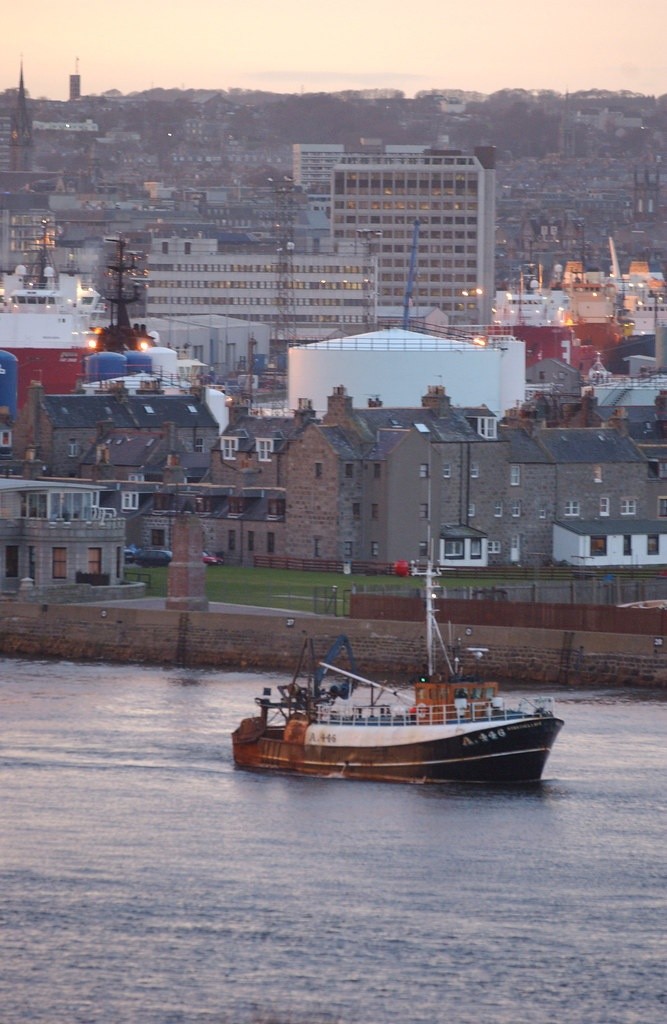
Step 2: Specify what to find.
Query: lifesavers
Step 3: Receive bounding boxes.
[417,703,427,718]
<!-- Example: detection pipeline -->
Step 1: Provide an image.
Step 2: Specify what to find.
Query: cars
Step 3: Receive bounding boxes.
[136,551,174,568]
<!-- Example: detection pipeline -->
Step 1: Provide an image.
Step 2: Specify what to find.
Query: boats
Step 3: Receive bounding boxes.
[203,551,224,567]
[229,438,566,785]
[124,545,135,564]
